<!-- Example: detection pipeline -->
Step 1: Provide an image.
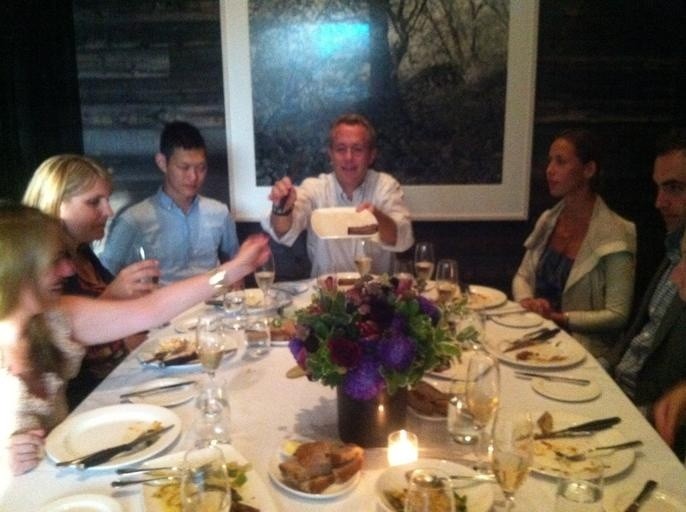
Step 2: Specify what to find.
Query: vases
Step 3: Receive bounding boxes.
[336,383,408,450]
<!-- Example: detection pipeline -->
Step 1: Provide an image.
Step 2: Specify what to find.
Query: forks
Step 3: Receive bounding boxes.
[554,439,643,463]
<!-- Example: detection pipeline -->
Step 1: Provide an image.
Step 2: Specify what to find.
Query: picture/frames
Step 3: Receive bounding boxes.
[219,0,540,222]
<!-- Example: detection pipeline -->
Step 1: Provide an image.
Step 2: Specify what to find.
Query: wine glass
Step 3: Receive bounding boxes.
[177,271,274,512]
[413,258,535,512]
[554,454,605,512]
[405,468,455,512]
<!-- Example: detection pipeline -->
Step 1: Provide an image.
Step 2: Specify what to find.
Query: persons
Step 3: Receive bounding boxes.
[649,230,685,465]
[100,121,249,292]
[20,156,162,415]
[598,139,686,419]
[260,113,415,276]
[512,132,638,359]
[0,199,271,477]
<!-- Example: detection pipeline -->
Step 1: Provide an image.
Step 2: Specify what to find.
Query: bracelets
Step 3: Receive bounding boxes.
[206,267,229,297]
[272,204,294,217]
[566,311,568,326]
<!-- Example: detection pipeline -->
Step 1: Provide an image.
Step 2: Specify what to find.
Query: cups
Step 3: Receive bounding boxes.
[131,245,161,287]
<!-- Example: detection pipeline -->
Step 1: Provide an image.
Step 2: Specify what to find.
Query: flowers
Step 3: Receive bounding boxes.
[286,272,483,402]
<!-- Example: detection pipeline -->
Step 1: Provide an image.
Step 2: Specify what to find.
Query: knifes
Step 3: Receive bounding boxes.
[622,481,659,511]
[513,370,592,387]
[120,379,197,398]
[535,416,622,440]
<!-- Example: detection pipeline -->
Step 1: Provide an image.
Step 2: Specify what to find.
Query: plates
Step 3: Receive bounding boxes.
[372,460,495,512]
[40,492,125,512]
[43,403,183,470]
[128,377,196,408]
[211,288,294,316]
[489,312,544,329]
[529,412,636,481]
[271,339,289,347]
[310,207,381,240]
[486,333,588,368]
[469,281,508,310]
[134,330,247,376]
[267,457,364,501]
[532,374,602,403]
[274,281,309,294]
